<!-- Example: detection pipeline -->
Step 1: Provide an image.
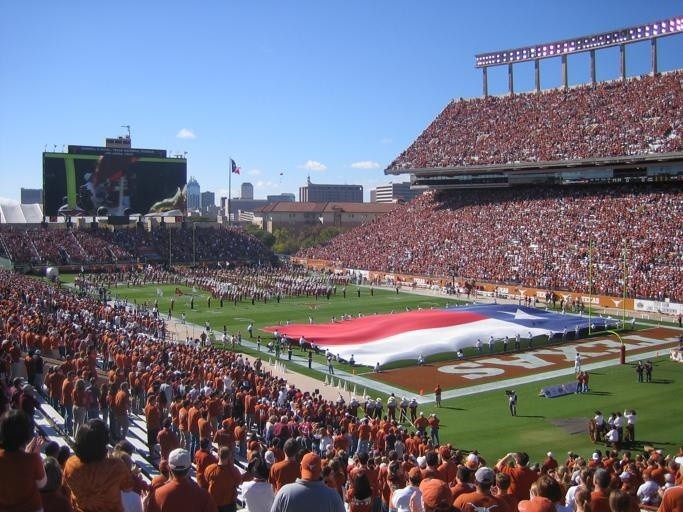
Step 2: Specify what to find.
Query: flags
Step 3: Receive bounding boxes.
[231,160,240,175]
[257,303,616,368]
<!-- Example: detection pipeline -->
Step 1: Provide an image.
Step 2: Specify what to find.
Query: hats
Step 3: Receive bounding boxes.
[439,447,450,458]
[326,443,334,451]
[13,377,24,385]
[431,414,436,417]
[345,412,350,418]
[2,340,9,344]
[193,384,197,388]
[258,400,262,402]
[474,467,494,484]
[420,412,424,416]
[35,349,41,354]
[272,401,276,407]
[656,450,663,454]
[168,448,191,470]
[472,450,480,454]
[402,396,406,401]
[416,456,426,468]
[300,452,322,479]
[352,398,356,401]
[368,399,373,404]
[467,460,475,468]
[397,425,403,429]
[675,456,683,464]
[66,355,71,359]
[419,478,453,510]
[366,396,371,399]
[222,418,232,426]
[262,397,266,402]
[593,452,599,460]
[146,366,151,371]
[265,451,275,464]
[207,381,213,384]
[437,385,440,387]
[53,365,61,371]
[412,398,416,401]
[546,452,552,457]
[407,467,422,478]
[416,431,422,436]
[22,384,34,391]
[376,398,381,402]
[664,473,673,481]
[618,472,630,478]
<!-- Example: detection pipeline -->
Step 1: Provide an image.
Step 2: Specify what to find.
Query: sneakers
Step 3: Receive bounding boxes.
[150,450,161,459]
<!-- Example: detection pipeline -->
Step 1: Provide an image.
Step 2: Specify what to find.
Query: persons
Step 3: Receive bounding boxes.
[608,489,629,512]
[464,450,480,470]
[503,335,508,352]
[204,445,242,512]
[488,336,494,353]
[528,331,532,348]
[268,438,302,492]
[514,333,520,352]
[391,466,421,512]
[240,461,276,512]
[582,371,588,392]
[574,325,578,339]
[574,485,591,512]
[376,424,388,449]
[492,473,517,512]
[364,395,371,414]
[589,469,611,512]
[604,426,621,452]
[387,393,396,422]
[592,410,605,443]
[150,460,170,492]
[401,453,414,471]
[344,473,373,512]
[636,470,660,507]
[427,412,440,448]
[378,450,398,512]
[613,412,623,444]
[0,409,47,512]
[360,413,368,424]
[476,338,482,353]
[398,397,407,423]
[270,452,346,512]
[366,400,375,417]
[661,474,675,490]
[416,352,424,366]
[331,460,346,506]
[650,457,669,484]
[394,434,405,460]
[574,352,581,373]
[418,438,428,456]
[195,438,219,491]
[323,467,335,491]
[536,474,572,512]
[357,418,370,452]
[146,449,217,511]
[619,472,633,495]
[391,420,397,432]
[565,467,593,507]
[677,315,682,327]
[607,412,616,430]
[405,431,419,456]
[409,477,458,512]
[575,371,583,393]
[420,452,444,483]
[546,329,553,344]
[408,399,417,423]
[452,467,502,512]
[543,449,655,480]
[368,420,377,448]
[109,452,141,512]
[414,411,428,435]
[372,398,384,421]
[372,361,384,374]
[657,456,682,512]
[644,360,652,382]
[495,451,539,498]
[414,430,421,446]
[623,407,635,442]
[507,389,517,416]
[517,497,556,512]
[379,416,388,431]
[383,428,396,452]
[437,447,457,483]
[114,441,135,457]
[563,326,567,341]
[435,384,441,408]
[348,452,378,497]
[457,347,464,359]
[635,360,643,382]
[388,461,404,511]
[420,431,427,443]
[0,258,360,459]
[449,468,473,500]
